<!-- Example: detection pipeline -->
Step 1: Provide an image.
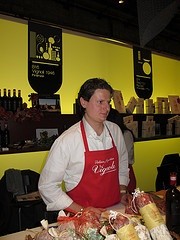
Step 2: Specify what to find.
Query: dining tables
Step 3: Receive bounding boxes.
[0,186,180,240]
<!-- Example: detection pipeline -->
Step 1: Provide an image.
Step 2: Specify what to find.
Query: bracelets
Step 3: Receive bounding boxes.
[119,189,126,193]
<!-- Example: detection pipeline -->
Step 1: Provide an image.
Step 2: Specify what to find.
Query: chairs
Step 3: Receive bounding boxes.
[155,153,180,192]
[0,168,59,234]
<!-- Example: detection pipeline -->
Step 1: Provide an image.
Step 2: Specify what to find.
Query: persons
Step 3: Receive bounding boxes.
[37,77,131,217]
[106,107,136,196]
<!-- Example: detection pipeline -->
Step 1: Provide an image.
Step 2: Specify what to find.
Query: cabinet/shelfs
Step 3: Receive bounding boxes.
[1,112,180,156]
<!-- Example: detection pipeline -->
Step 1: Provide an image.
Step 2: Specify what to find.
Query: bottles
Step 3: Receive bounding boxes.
[165,172,180,233]
[3,123,10,147]
[0,88,22,112]
[0,122,4,147]
